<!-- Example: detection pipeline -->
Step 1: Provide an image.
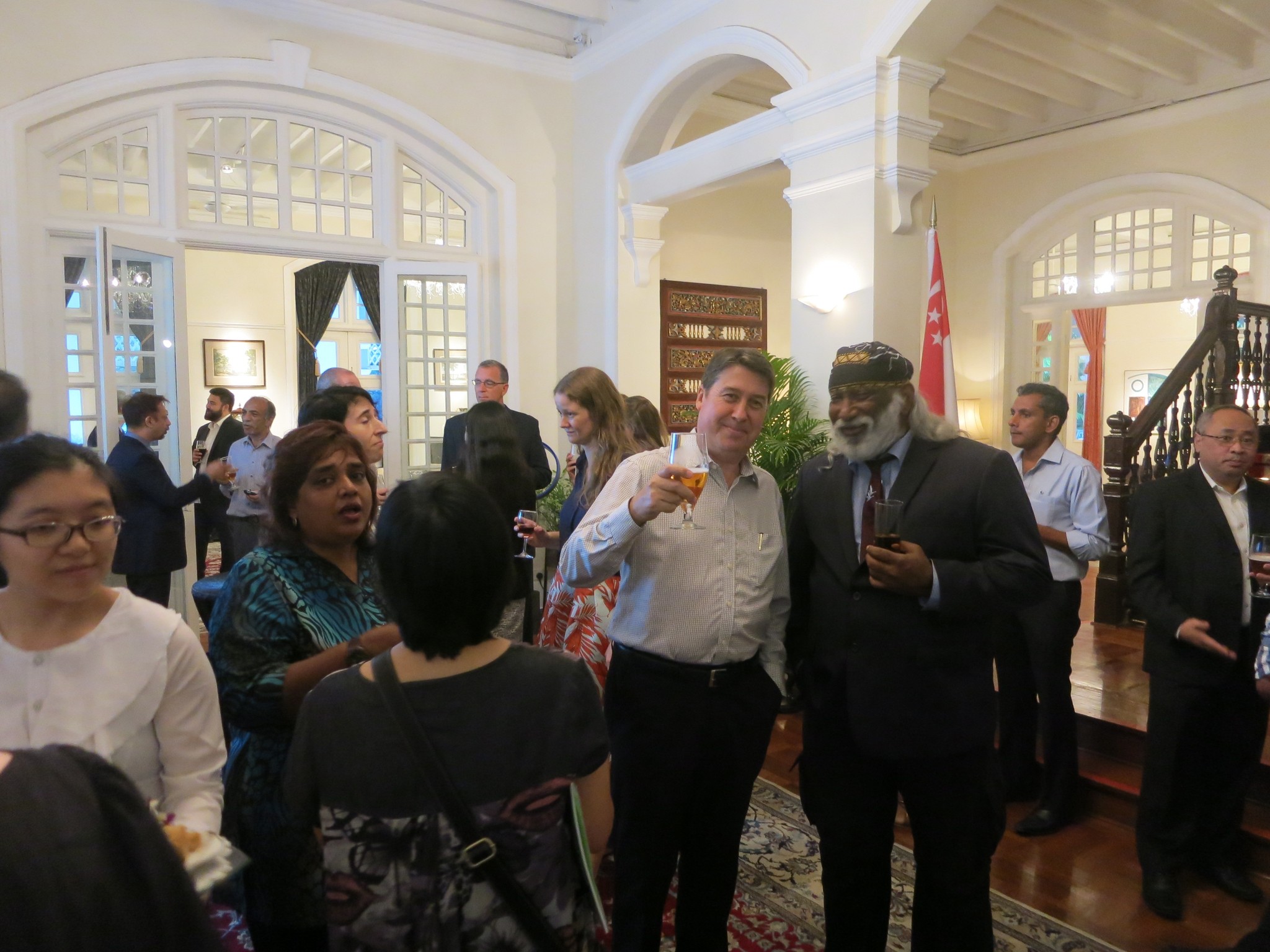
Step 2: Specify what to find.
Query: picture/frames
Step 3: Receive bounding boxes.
[433,349,468,392]
[202,339,266,388]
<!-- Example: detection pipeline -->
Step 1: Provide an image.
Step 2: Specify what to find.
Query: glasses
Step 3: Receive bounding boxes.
[472,379,505,388]
[1199,433,1259,449]
[1,514,125,550]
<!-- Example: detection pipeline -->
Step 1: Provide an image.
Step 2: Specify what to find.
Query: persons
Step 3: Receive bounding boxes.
[315,367,388,505]
[191,388,248,582]
[0,433,228,858]
[219,397,282,569]
[297,387,387,463]
[560,348,790,952]
[1254,612,1270,695]
[440,360,552,489]
[105,391,230,609]
[1128,403,1270,921]
[455,400,535,644]
[513,367,644,693]
[208,420,402,952]
[784,342,1054,952]
[1008,383,1112,836]
[285,472,614,952]
[625,396,668,451]
[87,391,126,448]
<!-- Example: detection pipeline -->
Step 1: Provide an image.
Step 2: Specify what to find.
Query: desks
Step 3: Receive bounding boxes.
[192,570,230,632]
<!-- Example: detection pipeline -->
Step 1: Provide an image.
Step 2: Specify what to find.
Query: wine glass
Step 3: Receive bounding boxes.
[569,444,581,483]
[667,433,710,529]
[515,510,538,559]
[1248,533,1270,598]
[220,456,241,491]
[196,440,207,463]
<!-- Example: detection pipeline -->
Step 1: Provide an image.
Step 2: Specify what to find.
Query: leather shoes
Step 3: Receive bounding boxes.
[1141,868,1182,921]
[1014,799,1086,836]
[1200,865,1262,902]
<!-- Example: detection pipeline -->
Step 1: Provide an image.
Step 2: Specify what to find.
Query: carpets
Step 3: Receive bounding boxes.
[205,773,1126,952]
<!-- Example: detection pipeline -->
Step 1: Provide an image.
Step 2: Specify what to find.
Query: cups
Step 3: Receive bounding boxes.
[873,500,904,554]
[375,474,386,496]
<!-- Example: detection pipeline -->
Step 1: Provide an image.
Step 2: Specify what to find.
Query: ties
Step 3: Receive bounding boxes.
[859,456,895,565]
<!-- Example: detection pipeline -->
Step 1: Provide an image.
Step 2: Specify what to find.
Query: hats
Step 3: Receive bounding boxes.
[829,342,914,398]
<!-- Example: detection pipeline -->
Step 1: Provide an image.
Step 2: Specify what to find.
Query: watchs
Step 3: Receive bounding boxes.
[343,638,367,669]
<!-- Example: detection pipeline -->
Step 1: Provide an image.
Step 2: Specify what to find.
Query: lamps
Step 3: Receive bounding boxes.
[222,164,233,173]
[403,191,465,300]
[111,264,154,315]
[204,201,232,214]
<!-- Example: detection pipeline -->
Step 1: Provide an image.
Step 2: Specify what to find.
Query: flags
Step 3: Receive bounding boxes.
[919,228,960,425]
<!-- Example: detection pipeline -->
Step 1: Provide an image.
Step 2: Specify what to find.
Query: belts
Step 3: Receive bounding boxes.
[612,643,761,689]
